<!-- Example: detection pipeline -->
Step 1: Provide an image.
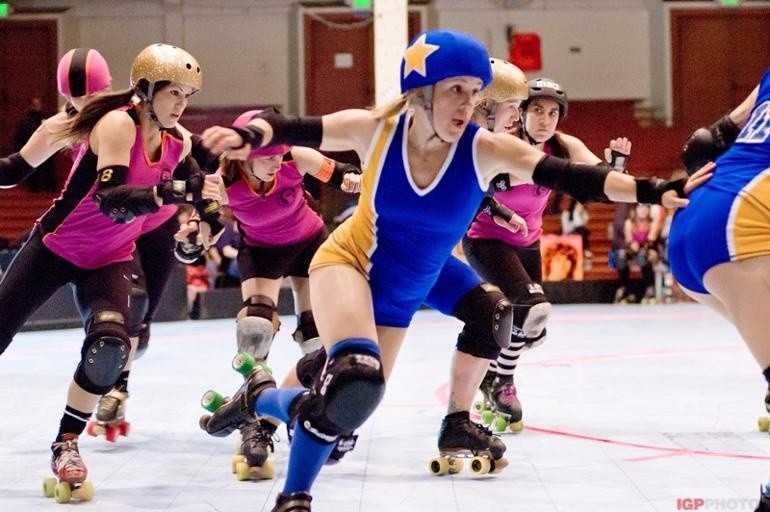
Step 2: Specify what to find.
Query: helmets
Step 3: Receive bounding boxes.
[232,110,290,159]
[129,43,203,97]
[399,29,492,89]
[476,55,529,105]
[55,47,113,98]
[521,77,568,119]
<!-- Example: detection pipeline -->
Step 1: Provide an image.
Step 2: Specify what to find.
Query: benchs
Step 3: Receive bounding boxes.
[173,104,280,137]
[3,174,66,245]
[533,96,702,281]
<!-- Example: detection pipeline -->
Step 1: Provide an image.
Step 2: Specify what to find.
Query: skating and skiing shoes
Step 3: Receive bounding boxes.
[473,370,525,435]
[88,387,132,444]
[231,422,273,480]
[199,352,279,438]
[41,434,97,505]
[430,412,510,479]
